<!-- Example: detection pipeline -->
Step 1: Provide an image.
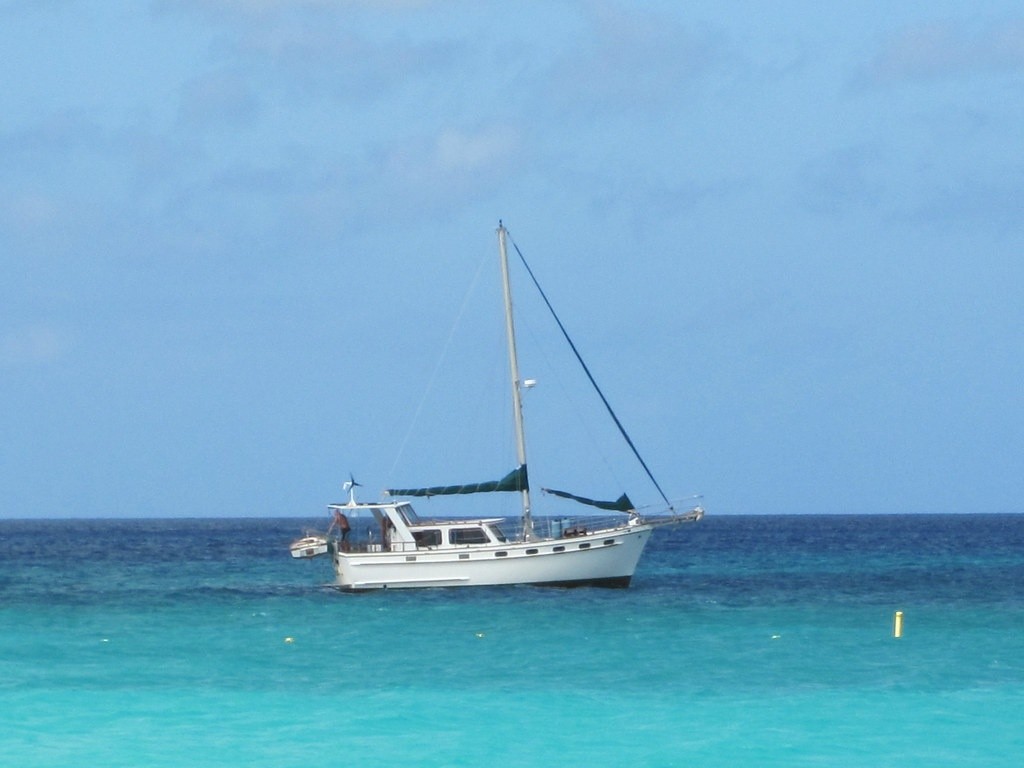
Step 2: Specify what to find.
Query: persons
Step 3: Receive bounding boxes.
[328,511,351,553]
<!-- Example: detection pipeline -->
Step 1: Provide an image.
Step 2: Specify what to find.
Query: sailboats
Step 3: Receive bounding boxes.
[289,219,706,594]
